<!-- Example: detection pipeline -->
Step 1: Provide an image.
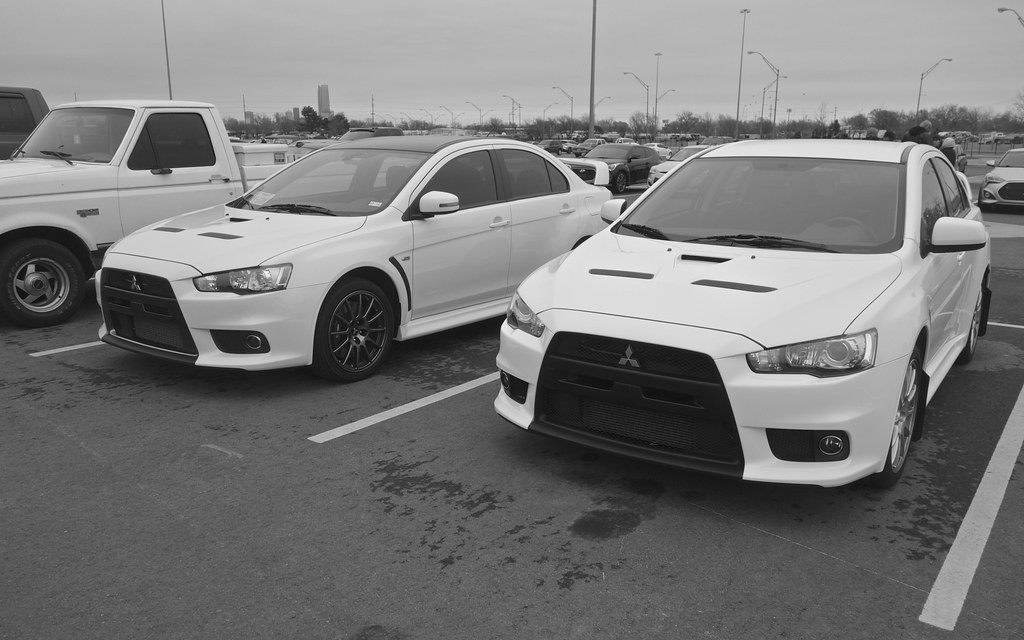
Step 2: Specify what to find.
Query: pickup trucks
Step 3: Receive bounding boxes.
[0,98,423,328]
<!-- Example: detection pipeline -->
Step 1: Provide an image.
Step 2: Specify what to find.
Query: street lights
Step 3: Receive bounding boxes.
[913,58,953,128]
[593,96,610,115]
[440,105,454,123]
[504,95,521,128]
[624,71,648,126]
[734,7,752,140]
[552,86,573,133]
[760,75,788,137]
[654,53,663,128]
[542,101,560,134]
[420,108,433,125]
[401,112,413,128]
[466,102,482,126]
[748,50,780,126]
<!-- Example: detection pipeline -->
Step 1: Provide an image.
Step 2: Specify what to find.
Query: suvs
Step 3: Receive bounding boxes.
[1,86,107,163]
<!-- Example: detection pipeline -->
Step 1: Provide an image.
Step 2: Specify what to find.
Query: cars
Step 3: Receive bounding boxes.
[491,138,992,491]
[228,126,1024,213]
[94,133,613,383]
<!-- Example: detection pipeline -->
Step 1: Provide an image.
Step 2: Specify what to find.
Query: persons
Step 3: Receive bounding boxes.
[901,120,934,146]
[883,132,894,141]
[940,137,956,168]
